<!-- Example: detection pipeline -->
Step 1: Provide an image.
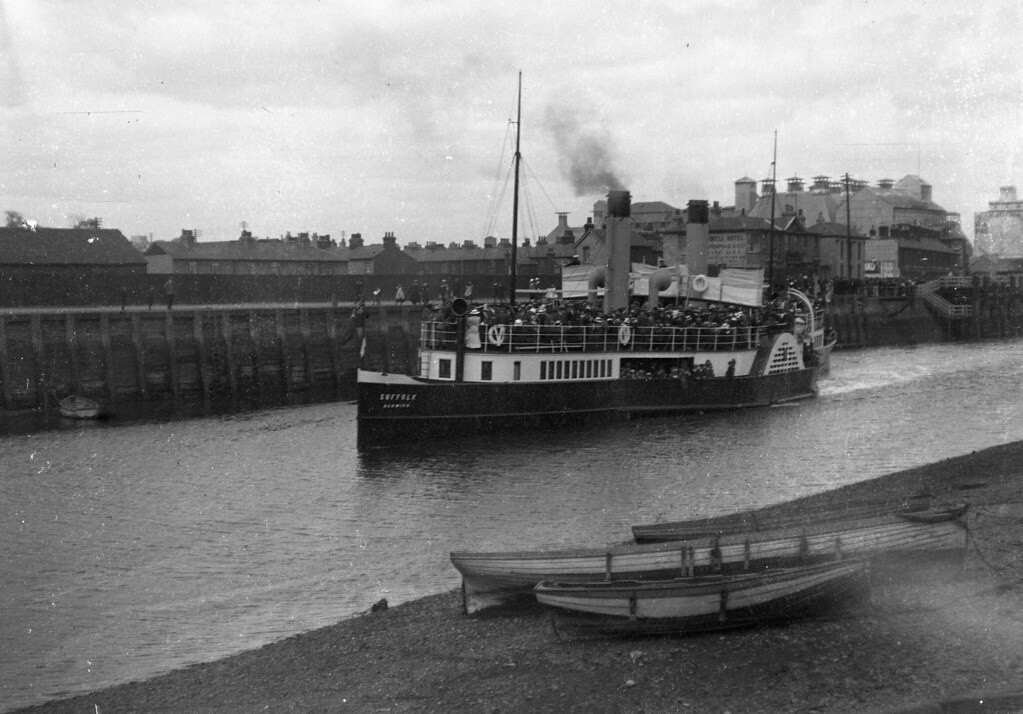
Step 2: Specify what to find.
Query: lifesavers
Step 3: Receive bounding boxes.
[488,324,506,345]
[618,325,631,345]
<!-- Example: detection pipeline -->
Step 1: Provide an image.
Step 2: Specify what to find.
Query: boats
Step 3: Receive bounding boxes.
[632,491,934,547]
[451,511,970,604]
[533,551,872,636]
[58,394,105,421]
[356,68,839,430]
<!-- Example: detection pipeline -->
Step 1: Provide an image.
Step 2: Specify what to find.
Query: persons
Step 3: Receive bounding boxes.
[120,284,129,311]
[420,274,924,350]
[726,358,736,376]
[394,284,406,305]
[408,280,421,304]
[619,360,715,382]
[372,287,383,305]
[353,278,364,304]
[163,276,178,310]
[147,288,155,310]
